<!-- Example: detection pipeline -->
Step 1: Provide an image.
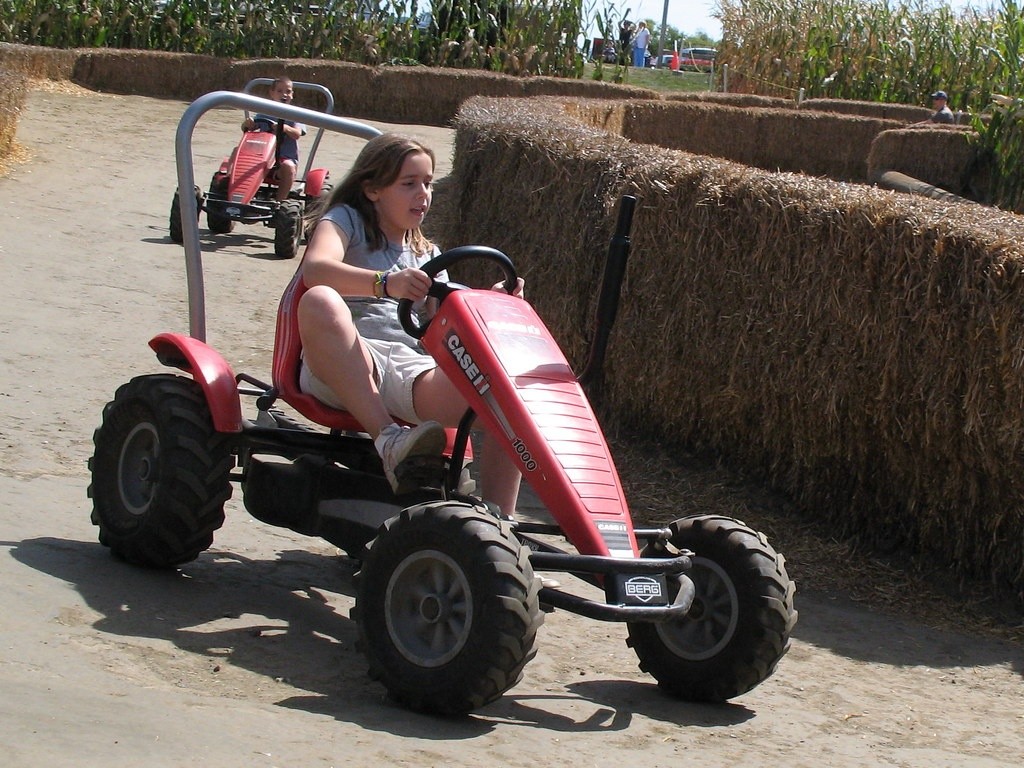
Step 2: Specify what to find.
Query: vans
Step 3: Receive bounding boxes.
[680,47,718,73]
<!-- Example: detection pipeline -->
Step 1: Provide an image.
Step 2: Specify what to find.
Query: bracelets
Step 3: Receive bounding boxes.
[373,270,394,299]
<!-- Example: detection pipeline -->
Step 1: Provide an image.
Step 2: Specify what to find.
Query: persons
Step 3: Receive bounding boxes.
[483,7,500,71]
[297,133,524,518]
[907,91,954,128]
[603,20,650,68]
[241,76,307,200]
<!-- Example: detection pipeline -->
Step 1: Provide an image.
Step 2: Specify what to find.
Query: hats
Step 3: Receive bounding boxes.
[931,91,947,101]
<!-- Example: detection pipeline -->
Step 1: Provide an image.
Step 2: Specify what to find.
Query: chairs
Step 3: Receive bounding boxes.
[274,231,418,432]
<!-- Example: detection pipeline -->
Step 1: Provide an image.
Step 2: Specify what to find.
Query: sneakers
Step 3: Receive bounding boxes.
[380,419,449,495]
[532,572,562,592]
